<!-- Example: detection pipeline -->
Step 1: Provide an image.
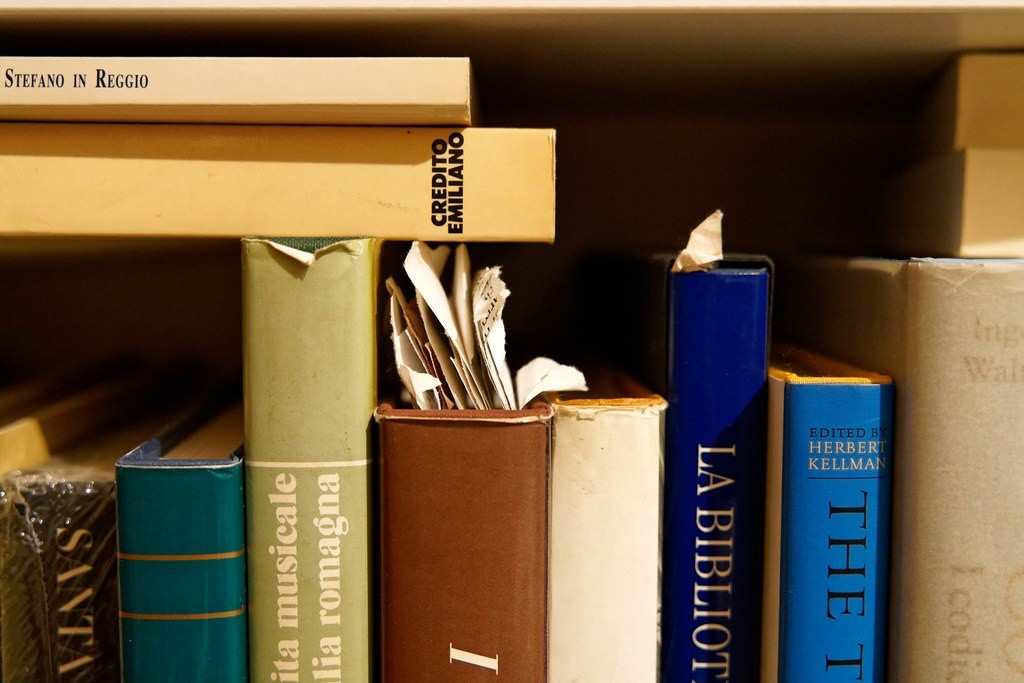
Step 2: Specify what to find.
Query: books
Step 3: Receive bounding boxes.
[0,56,1023,683]
